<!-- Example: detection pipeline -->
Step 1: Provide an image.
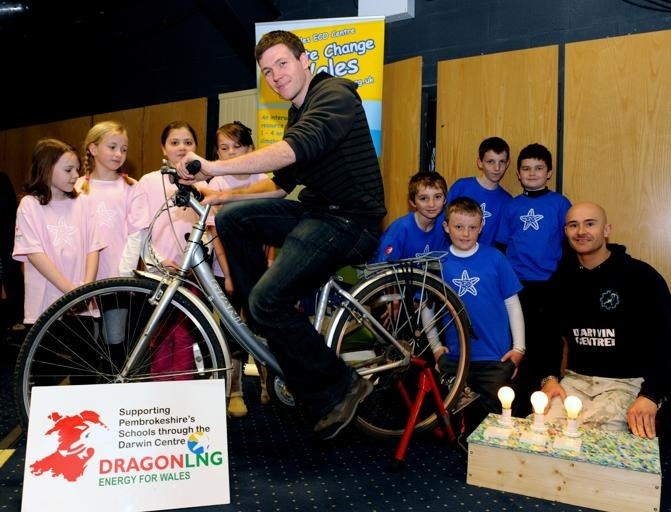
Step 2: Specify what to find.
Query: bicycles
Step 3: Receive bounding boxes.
[12,161,467,458]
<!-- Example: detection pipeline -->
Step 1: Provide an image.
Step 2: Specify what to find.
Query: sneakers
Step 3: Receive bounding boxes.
[227,392,247,417]
[313,378,372,440]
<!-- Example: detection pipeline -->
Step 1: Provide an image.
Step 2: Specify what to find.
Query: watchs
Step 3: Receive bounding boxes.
[541,375,559,387]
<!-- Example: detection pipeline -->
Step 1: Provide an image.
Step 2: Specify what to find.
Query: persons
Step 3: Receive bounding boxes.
[371,137,570,431]
[175,30,387,441]
[14,121,276,416]
[533,203,670,451]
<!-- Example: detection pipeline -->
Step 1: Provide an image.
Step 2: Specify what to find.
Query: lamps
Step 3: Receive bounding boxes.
[498,385,516,424]
[564,397,585,434]
[529,391,551,429]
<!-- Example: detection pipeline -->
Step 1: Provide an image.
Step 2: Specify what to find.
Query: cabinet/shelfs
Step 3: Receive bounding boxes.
[464,412,669,507]
